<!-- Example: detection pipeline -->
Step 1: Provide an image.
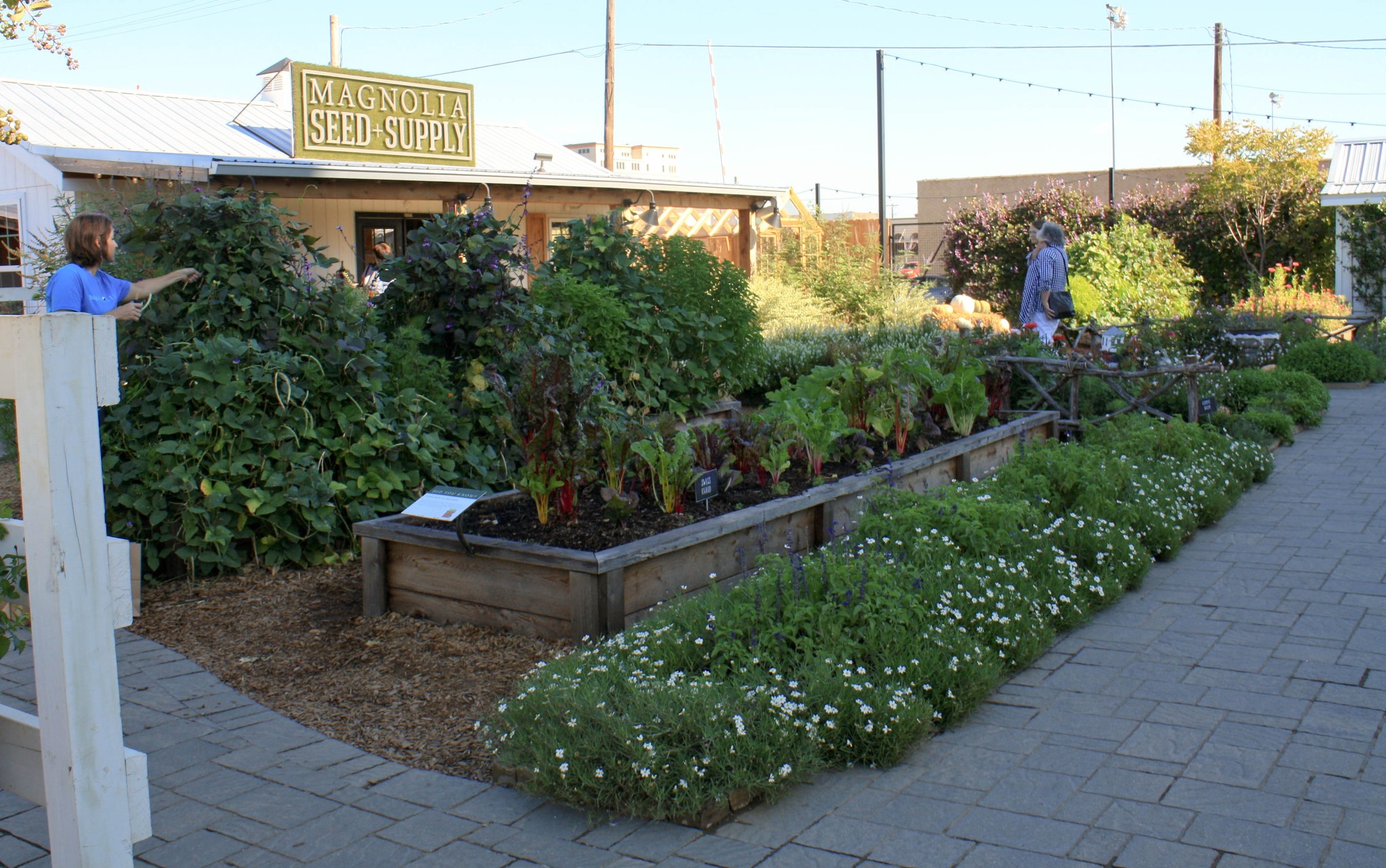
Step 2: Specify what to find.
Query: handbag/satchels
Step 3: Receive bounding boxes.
[1045,291,1074,319]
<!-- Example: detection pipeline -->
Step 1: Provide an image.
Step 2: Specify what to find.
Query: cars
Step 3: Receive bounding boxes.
[904,274,955,305]
[899,261,920,287]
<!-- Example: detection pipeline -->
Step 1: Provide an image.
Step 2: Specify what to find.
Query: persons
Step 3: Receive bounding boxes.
[1019,218,1068,359]
[46,211,201,320]
[360,242,397,310]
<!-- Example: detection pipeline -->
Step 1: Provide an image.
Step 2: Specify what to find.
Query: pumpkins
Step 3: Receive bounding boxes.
[998,319,1010,331]
[932,293,990,314]
